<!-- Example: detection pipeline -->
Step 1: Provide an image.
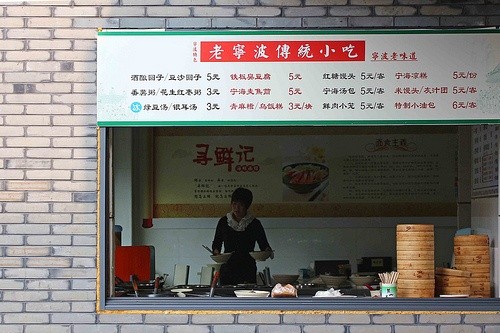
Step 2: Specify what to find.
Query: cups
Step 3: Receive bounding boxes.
[381,284,396,298]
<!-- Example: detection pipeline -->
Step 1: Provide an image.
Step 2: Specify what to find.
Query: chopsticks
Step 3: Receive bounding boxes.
[378,271,400,284]
[202,245,215,255]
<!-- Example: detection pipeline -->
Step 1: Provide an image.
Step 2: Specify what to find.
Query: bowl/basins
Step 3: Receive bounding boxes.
[350,273,377,287]
[210,253,233,263]
[320,274,348,289]
[282,163,330,194]
[249,251,273,261]
[234,289,270,298]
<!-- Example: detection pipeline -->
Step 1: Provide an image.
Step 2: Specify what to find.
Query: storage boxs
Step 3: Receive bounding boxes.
[357,257,392,272]
[315,260,350,278]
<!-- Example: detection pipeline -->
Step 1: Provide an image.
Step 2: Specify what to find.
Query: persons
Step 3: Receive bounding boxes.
[212,188,275,286]
